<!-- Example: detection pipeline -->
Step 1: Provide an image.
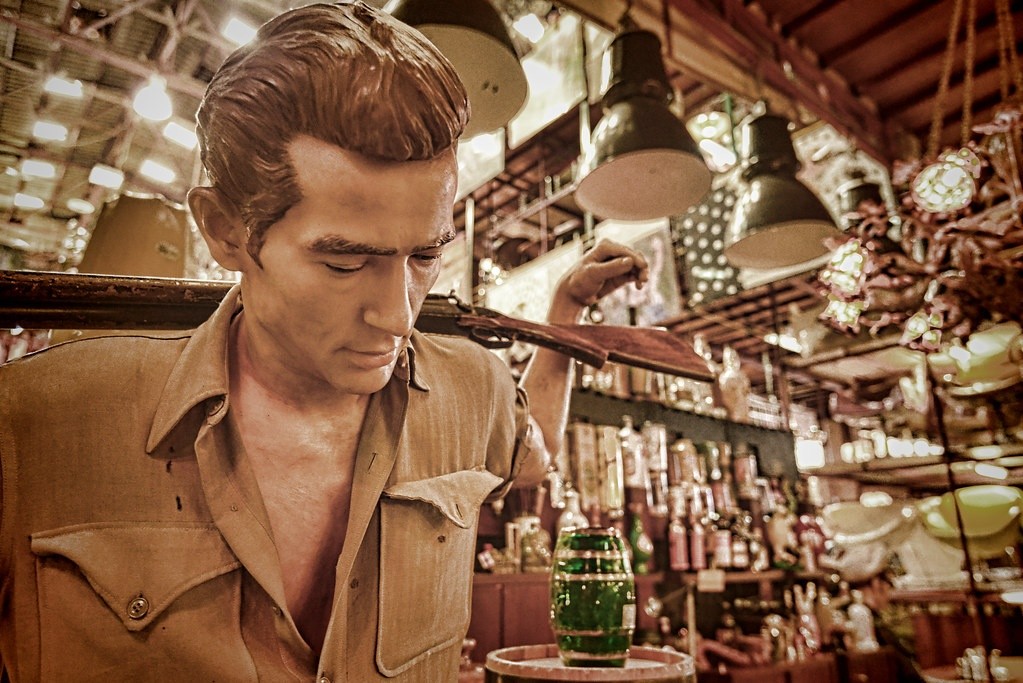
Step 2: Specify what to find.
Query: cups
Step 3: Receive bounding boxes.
[506,516,541,573]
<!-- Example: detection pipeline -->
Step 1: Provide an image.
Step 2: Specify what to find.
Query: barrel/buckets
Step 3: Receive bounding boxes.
[551,529,634,665]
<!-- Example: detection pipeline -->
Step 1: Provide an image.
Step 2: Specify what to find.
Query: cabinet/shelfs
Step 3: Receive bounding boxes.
[767,300,1023,683]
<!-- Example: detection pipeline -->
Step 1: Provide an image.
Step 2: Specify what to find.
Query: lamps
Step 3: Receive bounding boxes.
[133,73,173,120]
[573,11,713,222]
[381,0,532,144]
[723,97,846,270]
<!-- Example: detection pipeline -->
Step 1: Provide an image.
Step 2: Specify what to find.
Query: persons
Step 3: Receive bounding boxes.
[0,0,650,683]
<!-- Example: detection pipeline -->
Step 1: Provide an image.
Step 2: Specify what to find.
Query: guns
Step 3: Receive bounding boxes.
[0,265,716,385]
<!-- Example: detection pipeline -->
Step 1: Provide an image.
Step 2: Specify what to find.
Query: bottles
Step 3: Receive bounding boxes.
[522,523,552,573]
[849,589,878,652]
[556,492,589,534]
[620,334,769,574]
